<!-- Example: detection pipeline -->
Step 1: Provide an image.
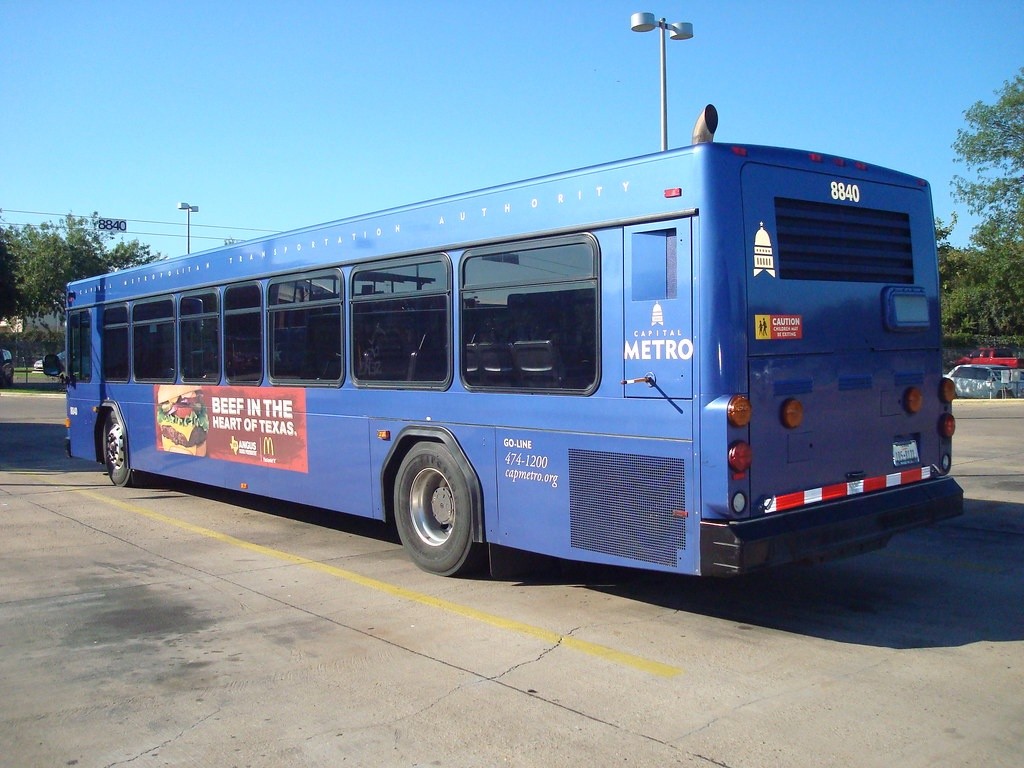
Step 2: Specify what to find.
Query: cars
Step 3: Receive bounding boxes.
[34,350,67,369]
[943,364,1024,400]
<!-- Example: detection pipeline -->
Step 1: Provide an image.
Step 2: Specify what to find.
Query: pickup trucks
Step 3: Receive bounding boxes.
[955,347,1024,369]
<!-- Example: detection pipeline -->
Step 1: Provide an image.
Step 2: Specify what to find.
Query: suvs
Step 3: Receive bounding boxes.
[0,347,15,387]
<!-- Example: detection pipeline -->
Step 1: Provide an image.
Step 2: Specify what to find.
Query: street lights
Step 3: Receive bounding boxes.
[630,12,695,150]
[177,202,199,255]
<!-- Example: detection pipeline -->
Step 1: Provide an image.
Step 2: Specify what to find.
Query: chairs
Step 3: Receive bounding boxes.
[191,350,215,377]
[275,341,306,378]
[444,341,560,388]
[407,350,433,381]
[359,348,385,378]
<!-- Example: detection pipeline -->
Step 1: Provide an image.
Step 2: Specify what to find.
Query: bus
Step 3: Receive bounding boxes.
[40,101,965,580]
